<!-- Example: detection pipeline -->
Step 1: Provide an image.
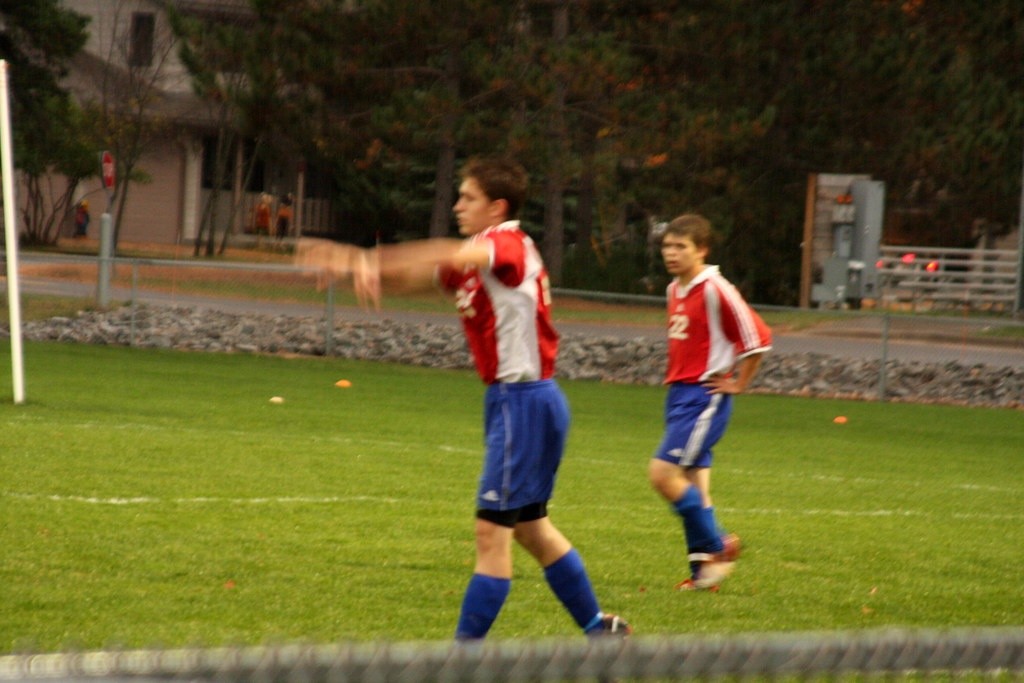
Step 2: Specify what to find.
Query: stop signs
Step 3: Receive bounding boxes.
[101,150,115,187]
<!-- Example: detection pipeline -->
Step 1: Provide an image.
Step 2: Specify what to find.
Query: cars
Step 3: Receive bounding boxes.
[875,251,941,305]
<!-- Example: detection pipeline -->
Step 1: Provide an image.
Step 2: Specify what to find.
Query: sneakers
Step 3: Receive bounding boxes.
[591,610,632,634]
[671,533,740,593]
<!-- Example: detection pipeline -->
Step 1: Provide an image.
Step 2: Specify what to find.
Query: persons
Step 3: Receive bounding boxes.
[255,192,274,251]
[647,213,773,590]
[274,193,293,252]
[290,153,630,644]
[72,198,93,240]
